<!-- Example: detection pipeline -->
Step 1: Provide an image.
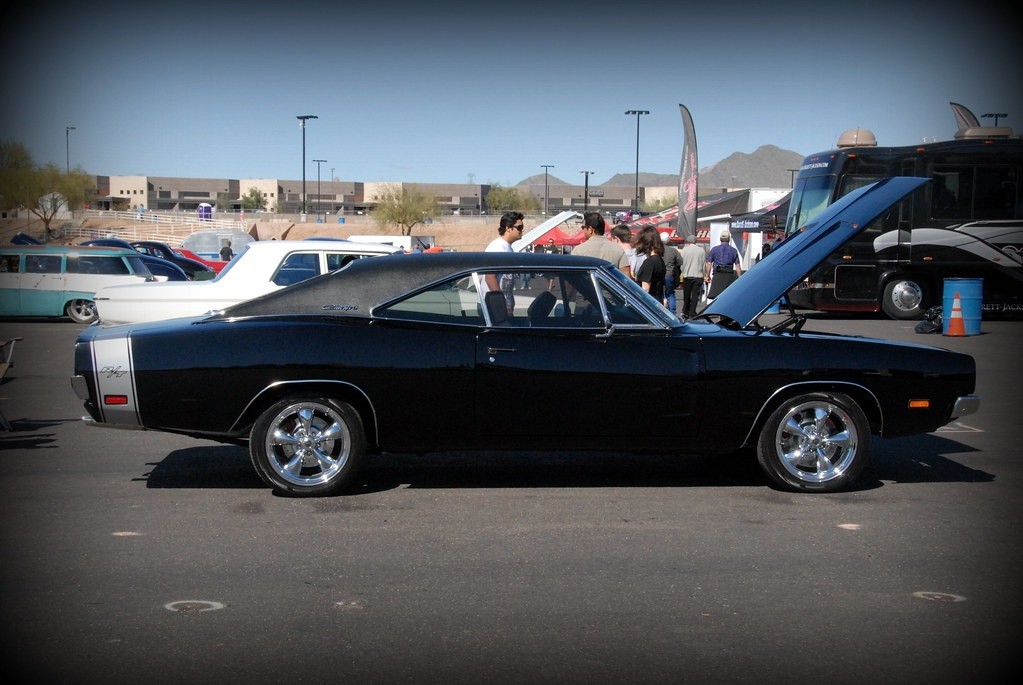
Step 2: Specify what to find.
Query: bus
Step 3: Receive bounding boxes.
[780,127,1023,321]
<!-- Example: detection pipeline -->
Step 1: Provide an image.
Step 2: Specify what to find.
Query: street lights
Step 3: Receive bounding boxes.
[66,126,76,173]
[981,113,1008,127]
[313,160,327,213]
[297,115,318,214]
[541,166,555,211]
[625,111,650,213]
[787,169,799,188]
[580,171,594,209]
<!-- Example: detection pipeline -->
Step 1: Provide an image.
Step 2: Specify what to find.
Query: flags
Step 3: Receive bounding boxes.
[676,104,699,243]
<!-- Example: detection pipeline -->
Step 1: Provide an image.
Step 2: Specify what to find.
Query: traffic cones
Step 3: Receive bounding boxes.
[943,292,970,337]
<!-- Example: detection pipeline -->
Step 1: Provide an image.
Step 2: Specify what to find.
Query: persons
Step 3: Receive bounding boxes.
[611,224,636,272]
[631,226,665,305]
[680,236,706,318]
[545,239,557,291]
[411,244,421,253]
[220,241,232,261]
[477,211,524,318]
[756,243,770,264]
[706,232,742,299]
[395,245,404,254]
[138,204,144,221]
[513,273,532,290]
[426,244,430,249]
[566,212,635,318]
[660,232,683,315]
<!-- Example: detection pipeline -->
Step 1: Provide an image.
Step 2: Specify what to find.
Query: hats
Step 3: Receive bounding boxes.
[720,232,730,241]
[659,232,670,242]
[687,235,697,243]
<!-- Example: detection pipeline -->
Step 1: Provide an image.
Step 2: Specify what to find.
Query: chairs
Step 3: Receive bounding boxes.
[0,337,24,432]
[484,290,557,328]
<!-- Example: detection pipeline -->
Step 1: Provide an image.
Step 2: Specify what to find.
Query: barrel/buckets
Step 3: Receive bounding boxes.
[339,218,345,223]
[942,277,985,336]
[763,295,786,314]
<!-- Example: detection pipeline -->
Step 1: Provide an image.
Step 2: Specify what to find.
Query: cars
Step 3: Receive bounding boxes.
[71,175,979,494]
[129,241,228,280]
[10,231,190,281]
[613,210,645,225]
[93,239,575,328]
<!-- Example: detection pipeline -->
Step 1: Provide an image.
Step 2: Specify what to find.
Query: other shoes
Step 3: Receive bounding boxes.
[521,286,524,290]
[513,287,517,291]
[528,286,532,291]
[552,285,555,290]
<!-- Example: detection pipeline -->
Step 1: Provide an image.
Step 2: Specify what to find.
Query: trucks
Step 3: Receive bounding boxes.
[347,235,435,252]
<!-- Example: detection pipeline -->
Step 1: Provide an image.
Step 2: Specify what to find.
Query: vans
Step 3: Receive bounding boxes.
[0,244,168,324]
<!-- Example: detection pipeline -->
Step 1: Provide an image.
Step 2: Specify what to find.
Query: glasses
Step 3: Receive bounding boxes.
[582,225,593,229]
[510,225,523,231]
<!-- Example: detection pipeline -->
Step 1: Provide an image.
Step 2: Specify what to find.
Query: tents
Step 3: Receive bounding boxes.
[532,226,571,253]
[562,221,612,255]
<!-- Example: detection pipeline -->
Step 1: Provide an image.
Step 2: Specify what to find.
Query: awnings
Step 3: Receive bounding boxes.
[729,190,794,232]
